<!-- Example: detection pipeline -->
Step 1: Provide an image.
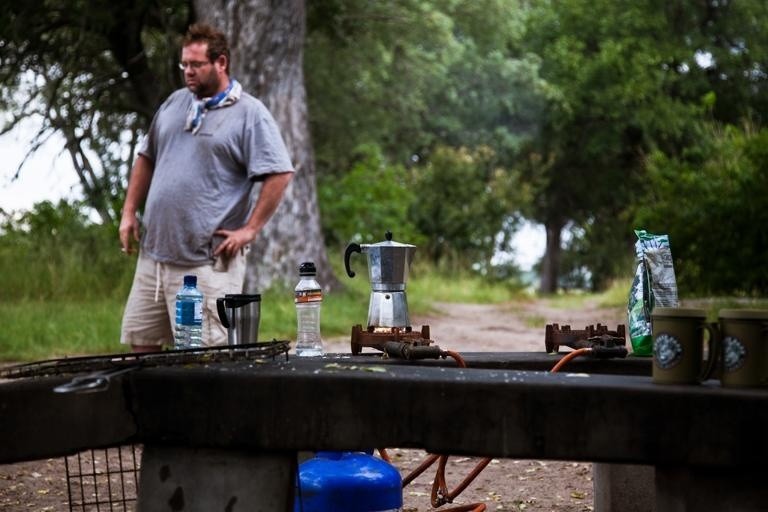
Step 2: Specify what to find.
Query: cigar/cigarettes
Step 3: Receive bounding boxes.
[120,247,137,253]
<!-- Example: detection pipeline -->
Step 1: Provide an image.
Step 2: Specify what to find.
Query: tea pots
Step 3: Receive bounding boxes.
[344,231,417,331]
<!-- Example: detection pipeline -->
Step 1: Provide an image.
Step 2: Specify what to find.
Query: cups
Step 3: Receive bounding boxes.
[216,291,263,348]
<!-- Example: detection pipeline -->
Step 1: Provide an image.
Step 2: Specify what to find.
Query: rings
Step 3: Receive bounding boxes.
[227,244,233,248]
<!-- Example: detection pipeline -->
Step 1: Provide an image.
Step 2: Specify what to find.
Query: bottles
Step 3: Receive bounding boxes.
[292,262,326,358]
[173,273,203,351]
[717,307,768,389]
[640,304,711,384]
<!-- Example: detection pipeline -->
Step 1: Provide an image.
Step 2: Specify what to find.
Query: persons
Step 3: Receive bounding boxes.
[118,22,297,355]
